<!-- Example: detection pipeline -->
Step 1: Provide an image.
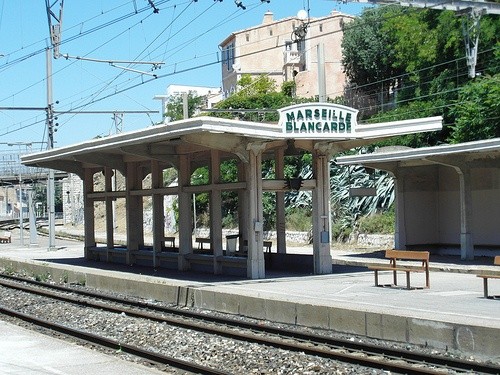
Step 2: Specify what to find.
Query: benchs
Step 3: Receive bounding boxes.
[0,231,12,244]
[476,255,500,299]
[367,249,432,290]
